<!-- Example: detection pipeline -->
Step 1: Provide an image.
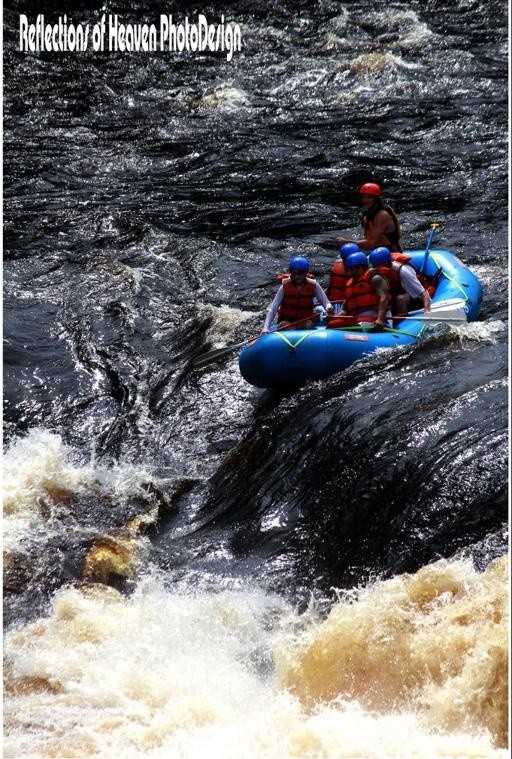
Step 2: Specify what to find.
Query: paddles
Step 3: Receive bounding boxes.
[393,297,464,315]
[192,312,327,367]
[328,308,468,328]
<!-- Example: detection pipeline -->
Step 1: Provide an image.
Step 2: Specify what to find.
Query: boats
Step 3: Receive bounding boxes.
[239,249,482,389]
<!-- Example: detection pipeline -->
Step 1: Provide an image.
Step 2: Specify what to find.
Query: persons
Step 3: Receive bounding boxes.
[256,183,433,335]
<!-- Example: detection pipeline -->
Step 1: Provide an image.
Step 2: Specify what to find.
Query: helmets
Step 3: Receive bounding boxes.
[345,252,368,267]
[370,247,391,265]
[359,184,381,196]
[341,244,361,258]
[290,257,309,271]
